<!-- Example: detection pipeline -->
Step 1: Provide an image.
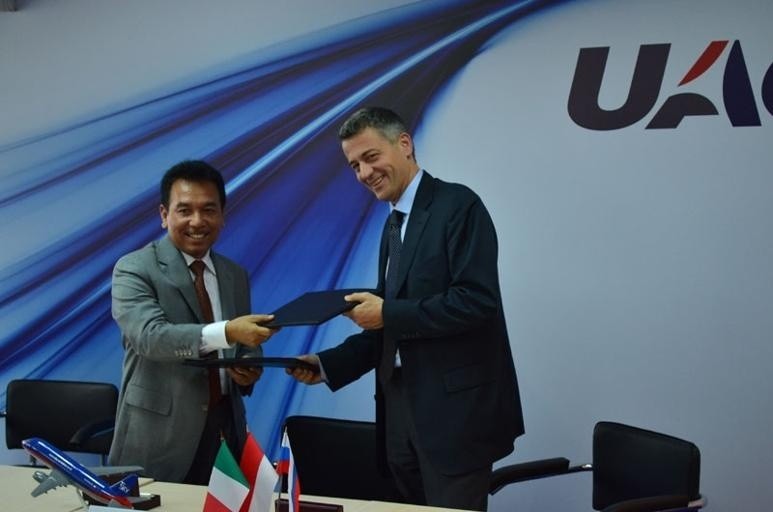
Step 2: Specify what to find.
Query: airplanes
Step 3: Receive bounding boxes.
[17,435,163,512]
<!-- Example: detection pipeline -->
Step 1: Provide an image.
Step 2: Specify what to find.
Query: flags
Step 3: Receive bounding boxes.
[200,436,251,512]
[273,429,301,512]
[238,430,280,512]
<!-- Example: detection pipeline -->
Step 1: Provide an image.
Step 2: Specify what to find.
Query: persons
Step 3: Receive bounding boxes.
[92,155,287,486]
[285,103,526,512]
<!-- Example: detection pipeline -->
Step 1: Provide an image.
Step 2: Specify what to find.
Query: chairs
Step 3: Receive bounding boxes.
[488,422,707,512]
[6,379,119,467]
[280,416,376,500]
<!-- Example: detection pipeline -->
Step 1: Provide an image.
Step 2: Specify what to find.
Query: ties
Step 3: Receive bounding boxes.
[187,259,224,407]
[379,210,405,378]
